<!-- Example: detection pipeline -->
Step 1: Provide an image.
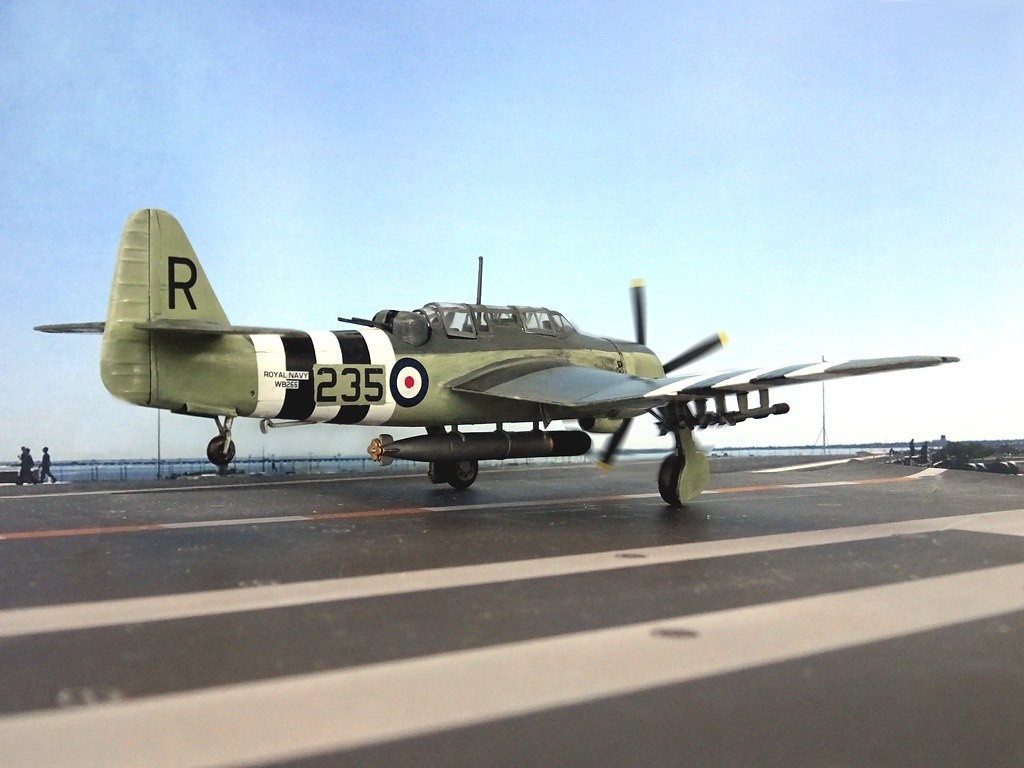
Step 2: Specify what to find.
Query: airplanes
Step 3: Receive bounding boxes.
[33,207,963,509]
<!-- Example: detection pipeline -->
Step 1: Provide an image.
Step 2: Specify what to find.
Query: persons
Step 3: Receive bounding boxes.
[15,445,40,486]
[908,438,914,456]
[39,447,57,483]
[920,442,928,458]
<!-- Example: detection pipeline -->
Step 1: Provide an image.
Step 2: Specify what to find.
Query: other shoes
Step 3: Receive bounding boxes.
[52,479,57,483]
[40,480,44,483]
